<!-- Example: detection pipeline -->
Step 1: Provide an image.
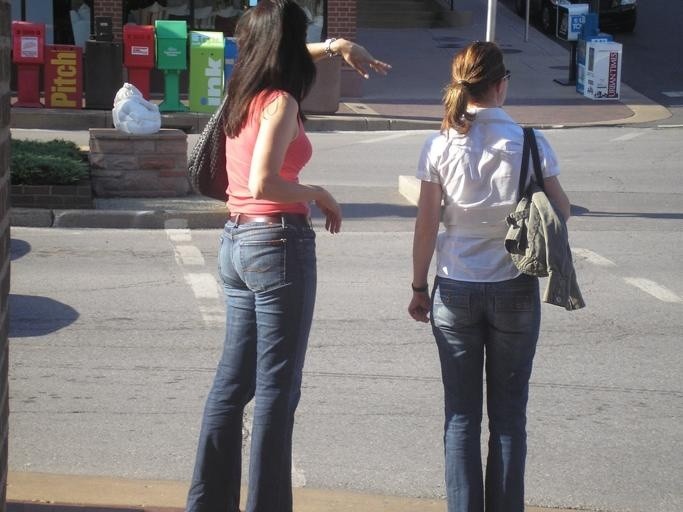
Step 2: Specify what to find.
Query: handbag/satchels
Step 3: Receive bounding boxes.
[186,96,230,202]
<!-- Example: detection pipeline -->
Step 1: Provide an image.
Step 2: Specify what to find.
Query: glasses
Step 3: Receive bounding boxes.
[495,69,512,84]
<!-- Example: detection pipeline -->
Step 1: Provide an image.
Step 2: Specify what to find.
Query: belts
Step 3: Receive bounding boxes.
[230,214,311,226]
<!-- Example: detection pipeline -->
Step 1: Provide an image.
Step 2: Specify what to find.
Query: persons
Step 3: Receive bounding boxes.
[403,42,573,512]
[183,0,394,511]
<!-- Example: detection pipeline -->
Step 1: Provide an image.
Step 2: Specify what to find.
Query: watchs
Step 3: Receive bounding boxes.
[323,36,338,58]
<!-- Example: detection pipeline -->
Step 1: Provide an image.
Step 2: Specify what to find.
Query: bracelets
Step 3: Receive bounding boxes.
[411,282,430,292]
